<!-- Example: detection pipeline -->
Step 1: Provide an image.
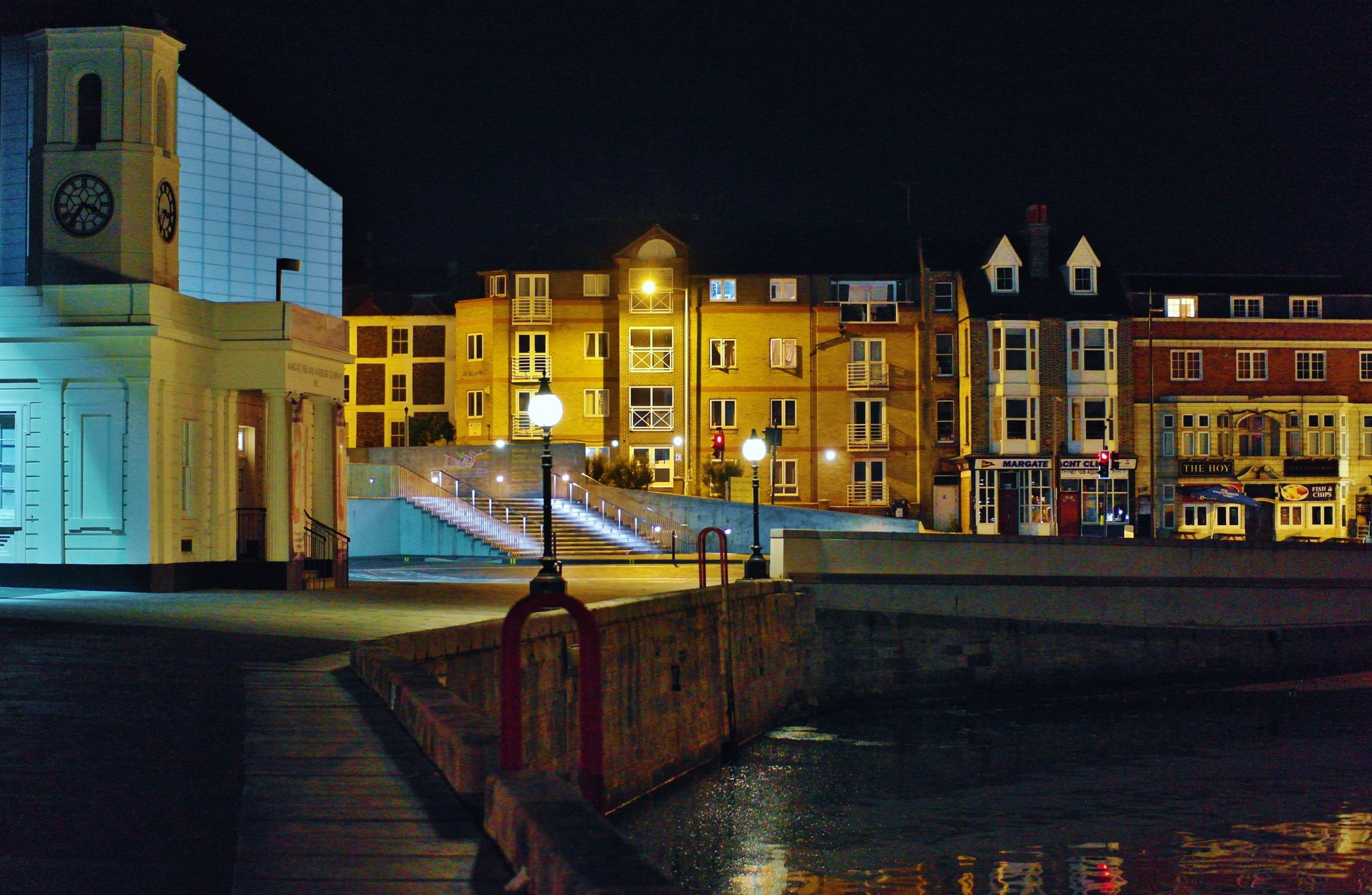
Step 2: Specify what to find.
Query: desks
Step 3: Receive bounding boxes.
[1216,533,1246,541]
[1331,537,1364,543]
[1167,531,1197,539]
[1291,536,1322,542]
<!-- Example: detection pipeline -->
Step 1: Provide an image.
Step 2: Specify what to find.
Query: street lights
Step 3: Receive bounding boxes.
[742,428,768,578]
[527,367,567,610]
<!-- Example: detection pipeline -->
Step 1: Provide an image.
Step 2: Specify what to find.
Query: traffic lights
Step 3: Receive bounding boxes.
[713,433,725,452]
[1097,450,1110,479]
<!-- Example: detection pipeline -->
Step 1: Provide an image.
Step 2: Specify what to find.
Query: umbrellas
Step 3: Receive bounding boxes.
[1175,483,1262,539]
